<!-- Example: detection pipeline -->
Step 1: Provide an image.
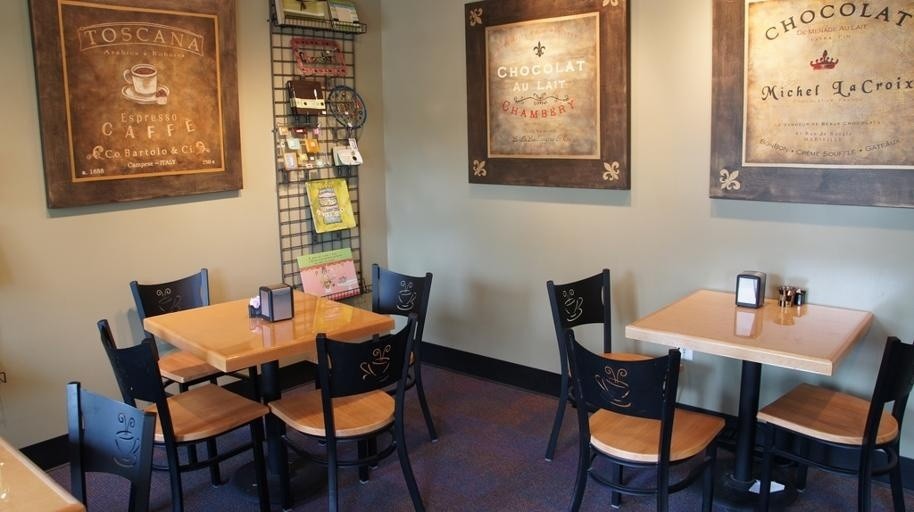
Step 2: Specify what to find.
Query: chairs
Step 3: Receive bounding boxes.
[757,336,914,512]
[95,319,269,512]
[314,263,439,469]
[268,312,426,512]
[65,381,157,512]
[544,268,655,462]
[565,329,726,512]
[128,267,265,472]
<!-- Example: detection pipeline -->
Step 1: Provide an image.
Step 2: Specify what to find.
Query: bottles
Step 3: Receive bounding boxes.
[794,306,805,318]
[795,289,806,306]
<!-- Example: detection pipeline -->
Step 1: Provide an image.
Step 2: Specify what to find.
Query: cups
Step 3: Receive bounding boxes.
[772,308,796,327]
[777,284,798,308]
[123,63,157,94]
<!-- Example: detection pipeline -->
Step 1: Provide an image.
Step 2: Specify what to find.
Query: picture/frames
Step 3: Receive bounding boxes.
[709,0,914,209]
[28,0,244,209]
[275,0,331,28]
[464,0,631,190]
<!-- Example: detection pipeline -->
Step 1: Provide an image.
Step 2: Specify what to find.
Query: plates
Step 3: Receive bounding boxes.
[121,84,170,105]
